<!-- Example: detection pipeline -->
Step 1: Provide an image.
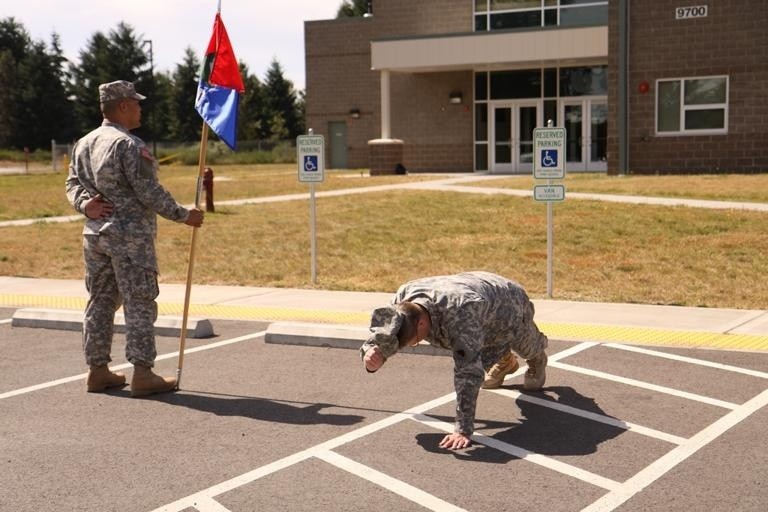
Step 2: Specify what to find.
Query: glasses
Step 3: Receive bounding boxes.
[410,331,420,348]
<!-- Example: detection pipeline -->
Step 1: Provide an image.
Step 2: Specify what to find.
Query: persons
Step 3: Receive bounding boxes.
[359,272,548,449]
[66,80,204,396]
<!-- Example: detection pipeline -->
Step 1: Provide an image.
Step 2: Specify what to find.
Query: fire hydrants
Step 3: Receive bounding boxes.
[203,168,215,213]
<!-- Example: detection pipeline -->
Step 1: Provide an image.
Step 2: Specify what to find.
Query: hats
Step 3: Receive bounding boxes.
[370,306,403,356]
[100,79,146,102]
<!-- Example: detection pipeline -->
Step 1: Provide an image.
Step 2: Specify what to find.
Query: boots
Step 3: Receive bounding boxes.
[87,366,126,394]
[481,351,519,390]
[131,365,177,395]
[523,350,546,391]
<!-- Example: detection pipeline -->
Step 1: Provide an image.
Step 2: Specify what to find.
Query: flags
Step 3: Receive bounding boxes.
[196,15,245,152]
